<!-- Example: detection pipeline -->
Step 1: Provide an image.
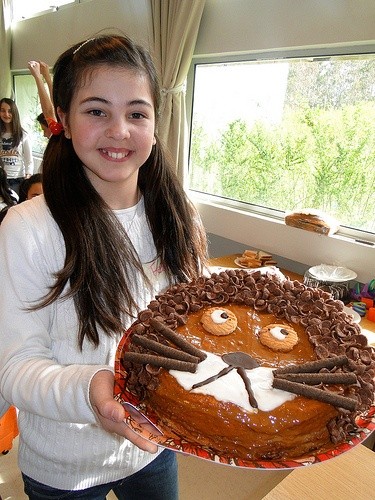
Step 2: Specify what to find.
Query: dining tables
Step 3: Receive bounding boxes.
[206,253,375,349]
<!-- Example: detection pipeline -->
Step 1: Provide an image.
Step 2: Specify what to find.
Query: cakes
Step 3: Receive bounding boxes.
[122,265,375,461]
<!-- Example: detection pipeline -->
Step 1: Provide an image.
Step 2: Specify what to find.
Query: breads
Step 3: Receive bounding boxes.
[238,249,278,268]
[285,212,330,235]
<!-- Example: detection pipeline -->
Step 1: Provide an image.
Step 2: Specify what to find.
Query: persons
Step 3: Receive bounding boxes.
[0,168,19,211]
[37,113,53,139]
[28,59,62,139]
[0,98,34,194]
[0,174,43,224]
[0,34,208,500]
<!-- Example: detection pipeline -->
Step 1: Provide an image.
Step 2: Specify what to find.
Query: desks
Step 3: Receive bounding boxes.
[261,445,375,500]
